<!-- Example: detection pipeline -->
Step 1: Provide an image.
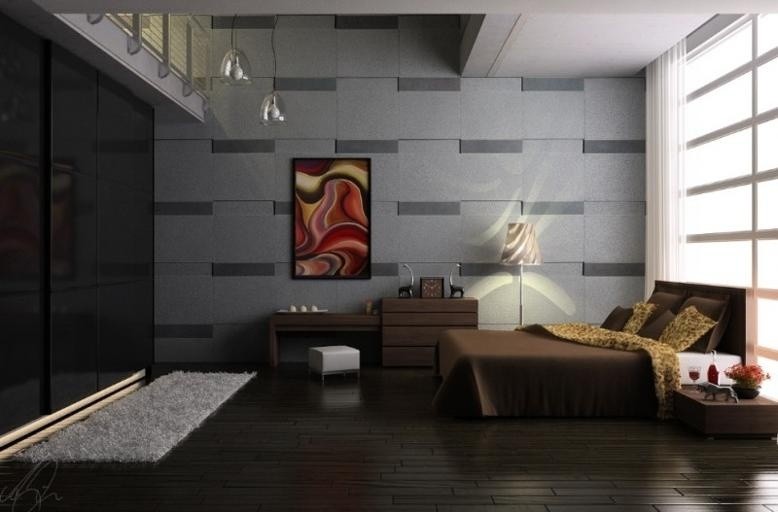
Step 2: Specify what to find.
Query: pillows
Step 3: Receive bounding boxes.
[678,290,735,353]
[658,305,719,352]
[638,308,677,340]
[600,305,633,331]
[647,285,687,313]
[622,302,657,334]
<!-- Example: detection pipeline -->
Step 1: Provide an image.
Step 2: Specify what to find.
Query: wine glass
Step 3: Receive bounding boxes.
[688,364,701,394]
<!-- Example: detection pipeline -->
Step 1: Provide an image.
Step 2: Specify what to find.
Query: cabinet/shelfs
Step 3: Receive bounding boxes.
[268,313,382,368]
[382,297,478,367]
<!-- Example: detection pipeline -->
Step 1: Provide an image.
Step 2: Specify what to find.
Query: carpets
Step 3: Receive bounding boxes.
[9,367,259,464]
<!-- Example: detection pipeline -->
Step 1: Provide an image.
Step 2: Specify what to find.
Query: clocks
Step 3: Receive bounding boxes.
[421,277,444,299]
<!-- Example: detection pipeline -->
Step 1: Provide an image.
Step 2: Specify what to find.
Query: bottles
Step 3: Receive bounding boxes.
[372,304,377,316]
[707,351,718,385]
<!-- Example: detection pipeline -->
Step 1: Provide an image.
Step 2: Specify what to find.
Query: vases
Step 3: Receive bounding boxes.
[731,384,759,400]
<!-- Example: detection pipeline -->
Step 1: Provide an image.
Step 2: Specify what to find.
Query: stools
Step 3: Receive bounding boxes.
[308,345,361,386]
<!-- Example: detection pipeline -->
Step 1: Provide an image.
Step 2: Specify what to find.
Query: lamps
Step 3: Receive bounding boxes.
[498,223,541,326]
[259,14,288,126]
[219,14,254,88]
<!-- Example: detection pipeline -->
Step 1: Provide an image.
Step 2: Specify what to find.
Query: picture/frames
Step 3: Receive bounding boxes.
[291,157,372,280]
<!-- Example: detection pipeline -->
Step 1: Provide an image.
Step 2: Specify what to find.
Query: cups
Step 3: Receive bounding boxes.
[300,305,307,312]
[312,305,318,312]
[290,305,296,312]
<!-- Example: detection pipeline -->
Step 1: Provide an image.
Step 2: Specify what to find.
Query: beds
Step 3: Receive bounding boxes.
[432,280,756,420]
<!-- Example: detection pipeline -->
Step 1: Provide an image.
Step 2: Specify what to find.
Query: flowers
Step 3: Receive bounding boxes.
[723,362,771,390]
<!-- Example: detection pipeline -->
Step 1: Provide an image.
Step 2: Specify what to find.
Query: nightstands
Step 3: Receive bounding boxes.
[671,385,778,441]
